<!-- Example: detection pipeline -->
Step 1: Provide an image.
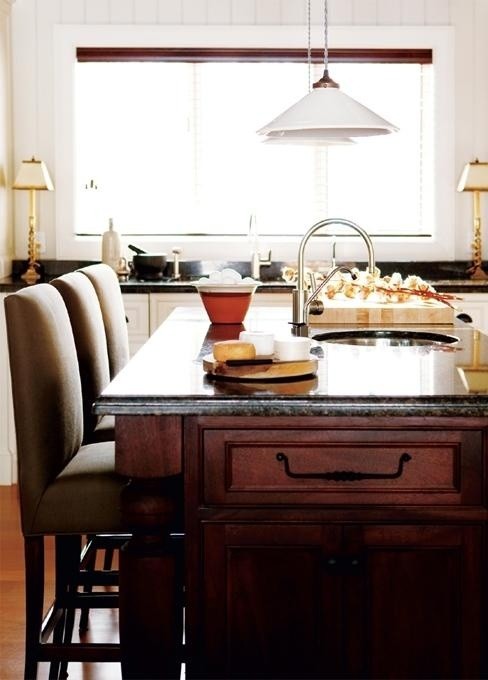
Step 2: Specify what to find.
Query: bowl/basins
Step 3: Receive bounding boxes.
[192,282,262,325]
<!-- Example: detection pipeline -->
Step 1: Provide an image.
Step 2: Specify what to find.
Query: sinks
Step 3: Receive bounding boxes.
[314,329,459,347]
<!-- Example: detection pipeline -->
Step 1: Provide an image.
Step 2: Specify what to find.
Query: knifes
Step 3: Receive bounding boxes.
[226,356,308,366]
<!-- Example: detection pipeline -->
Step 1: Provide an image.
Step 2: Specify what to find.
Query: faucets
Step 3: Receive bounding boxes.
[293,219,375,327]
[248,214,272,280]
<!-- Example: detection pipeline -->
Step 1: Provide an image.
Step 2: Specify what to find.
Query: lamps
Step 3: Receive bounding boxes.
[12,156,55,285]
[440,293,487,334]
[457,157,488,280]
[255,1,400,145]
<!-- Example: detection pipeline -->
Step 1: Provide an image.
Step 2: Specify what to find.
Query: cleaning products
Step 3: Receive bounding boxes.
[101,217,121,272]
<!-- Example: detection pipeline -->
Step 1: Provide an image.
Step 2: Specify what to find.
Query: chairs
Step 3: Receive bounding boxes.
[2,263,134,676]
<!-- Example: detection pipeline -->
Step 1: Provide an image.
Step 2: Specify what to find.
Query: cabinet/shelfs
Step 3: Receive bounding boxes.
[181,416,487,680]
[122,293,203,362]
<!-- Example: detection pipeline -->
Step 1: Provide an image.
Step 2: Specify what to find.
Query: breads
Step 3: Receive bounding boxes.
[212,339,257,362]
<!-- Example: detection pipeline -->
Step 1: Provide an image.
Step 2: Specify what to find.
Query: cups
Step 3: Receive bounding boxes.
[272,335,315,361]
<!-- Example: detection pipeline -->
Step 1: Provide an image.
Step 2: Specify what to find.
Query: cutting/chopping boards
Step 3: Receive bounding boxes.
[203,352,320,380]
[306,291,455,325]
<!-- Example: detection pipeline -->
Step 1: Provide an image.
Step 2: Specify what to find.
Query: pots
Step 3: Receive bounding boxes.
[128,245,204,279]
